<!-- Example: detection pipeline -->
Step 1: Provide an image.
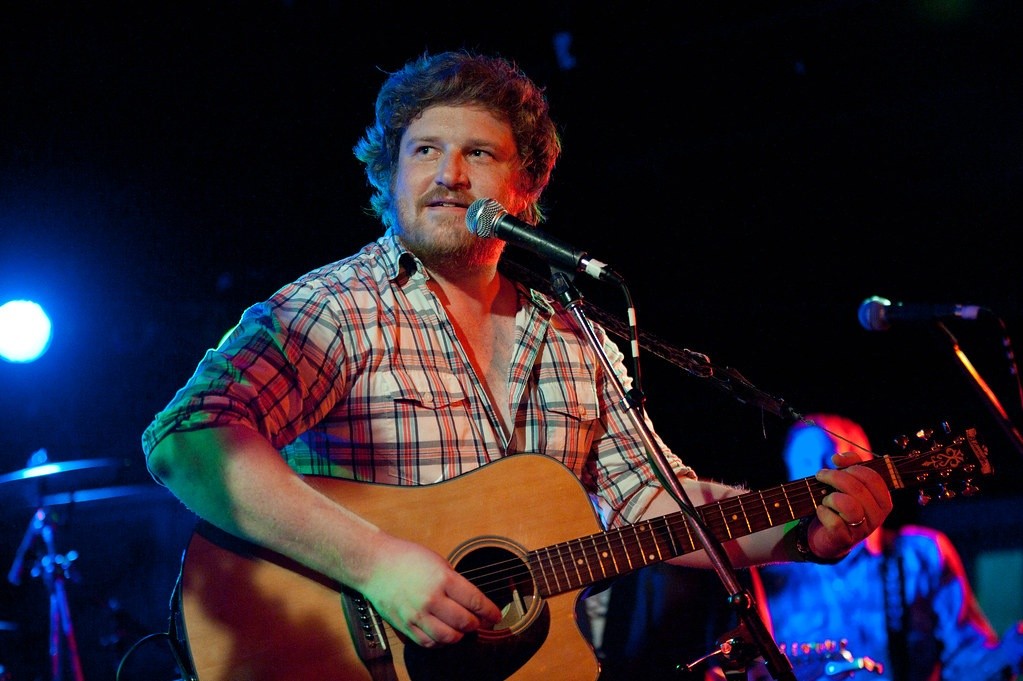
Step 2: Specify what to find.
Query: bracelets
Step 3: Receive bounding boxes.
[797,520,846,565]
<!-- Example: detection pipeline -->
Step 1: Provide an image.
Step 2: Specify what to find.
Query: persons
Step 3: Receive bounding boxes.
[731,417,1002,680]
[142,48,896,647]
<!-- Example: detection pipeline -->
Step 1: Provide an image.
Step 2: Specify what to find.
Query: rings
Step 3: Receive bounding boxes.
[850,516,865,526]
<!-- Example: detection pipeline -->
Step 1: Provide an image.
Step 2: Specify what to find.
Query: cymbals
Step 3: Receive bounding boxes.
[0,457,122,482]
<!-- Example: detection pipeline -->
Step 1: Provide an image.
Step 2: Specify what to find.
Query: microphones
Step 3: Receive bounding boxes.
[859,296,993,330]
[466,197,623,282]
[8,510,49,586]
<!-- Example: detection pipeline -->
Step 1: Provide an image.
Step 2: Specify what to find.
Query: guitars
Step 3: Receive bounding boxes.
[172,415,996,681]
